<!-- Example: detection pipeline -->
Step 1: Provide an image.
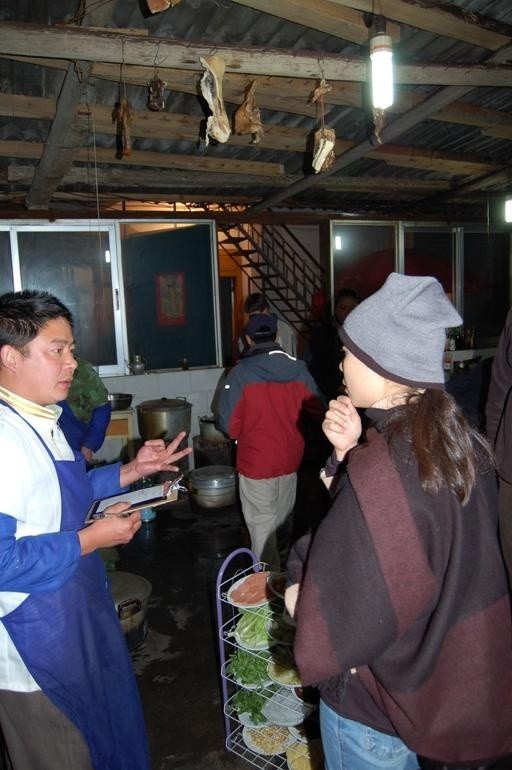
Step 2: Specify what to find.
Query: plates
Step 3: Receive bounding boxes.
[227,570,322,770]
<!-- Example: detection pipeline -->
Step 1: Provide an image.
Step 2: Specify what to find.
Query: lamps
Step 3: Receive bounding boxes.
[368,15,394,112]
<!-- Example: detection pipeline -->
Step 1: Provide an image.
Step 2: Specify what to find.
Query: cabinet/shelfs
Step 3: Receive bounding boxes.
[216,545,325,770]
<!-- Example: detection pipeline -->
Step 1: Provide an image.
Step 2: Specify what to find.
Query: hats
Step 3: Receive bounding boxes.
[336,273,463,393]
[244,312,278,336]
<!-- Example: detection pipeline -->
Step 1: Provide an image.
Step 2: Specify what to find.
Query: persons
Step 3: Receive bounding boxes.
[485,311,511,570]
[55,352,123,573]
[0,289,195,770]
[273,264,511,770]
[237,293,297,359]
[313,288,366,402]
[213,311,324,573]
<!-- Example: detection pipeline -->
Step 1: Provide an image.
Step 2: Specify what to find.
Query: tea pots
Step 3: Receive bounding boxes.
[124,355,147,375]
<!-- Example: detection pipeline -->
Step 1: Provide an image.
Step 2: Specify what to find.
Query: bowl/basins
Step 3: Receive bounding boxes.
[108,393,133,410]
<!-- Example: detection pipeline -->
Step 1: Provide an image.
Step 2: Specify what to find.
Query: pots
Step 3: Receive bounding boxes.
[106,572,155,652]
[188,464,237,514]
[136,396,193,441]
[197,415,225,440]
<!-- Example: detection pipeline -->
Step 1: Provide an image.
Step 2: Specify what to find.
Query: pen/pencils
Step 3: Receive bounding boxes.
[93,513,130,519]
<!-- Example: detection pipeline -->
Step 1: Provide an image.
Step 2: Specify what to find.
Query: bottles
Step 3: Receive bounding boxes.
[134,476,157,524]
